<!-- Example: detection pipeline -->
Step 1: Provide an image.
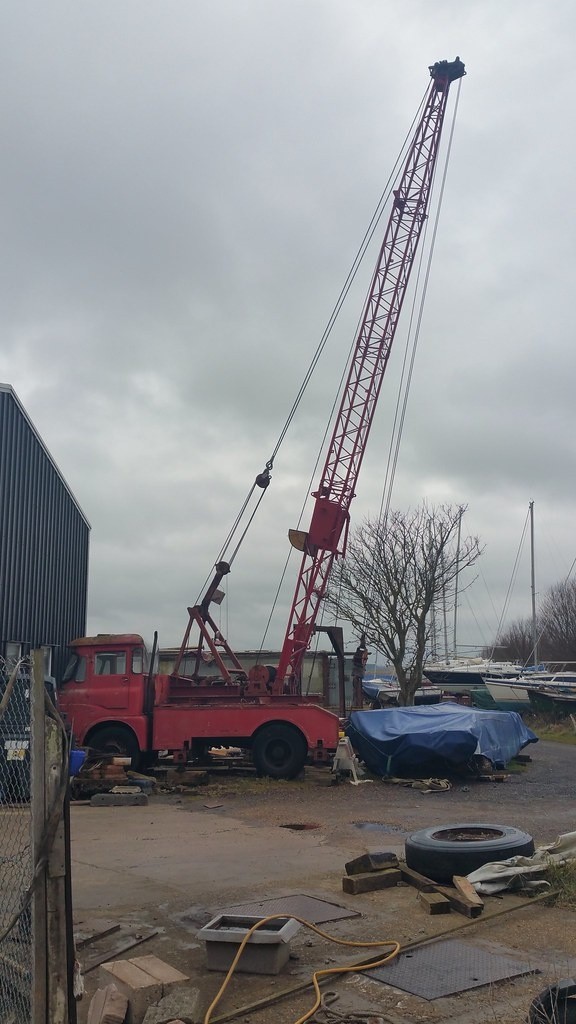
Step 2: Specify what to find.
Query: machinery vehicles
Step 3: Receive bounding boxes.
[55,56,465,780]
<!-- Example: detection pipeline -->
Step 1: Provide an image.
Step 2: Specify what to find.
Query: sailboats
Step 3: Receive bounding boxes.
[364,500,548,703]
[482,557,576,704]
